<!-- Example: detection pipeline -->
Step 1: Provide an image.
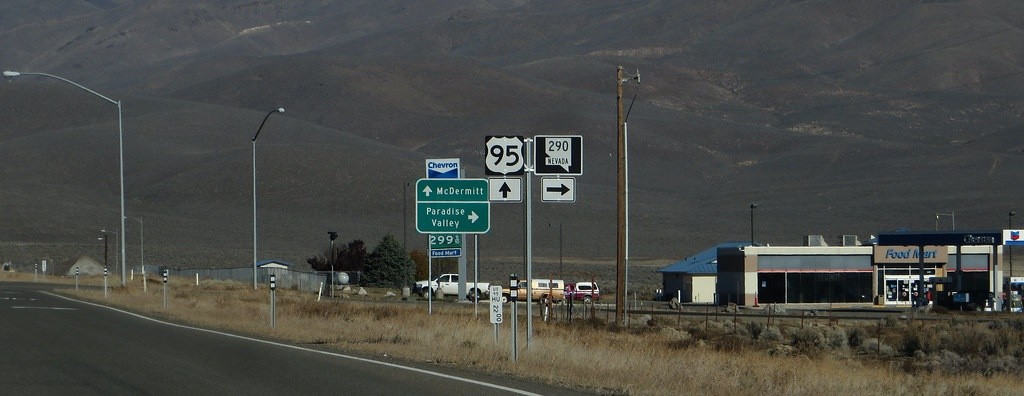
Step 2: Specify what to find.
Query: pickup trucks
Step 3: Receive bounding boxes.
[565,280,600,304]
[501,277,566,304]
[415,272,491,302]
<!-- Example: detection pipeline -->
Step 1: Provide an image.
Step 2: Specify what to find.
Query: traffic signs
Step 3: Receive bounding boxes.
[415,177,491,234]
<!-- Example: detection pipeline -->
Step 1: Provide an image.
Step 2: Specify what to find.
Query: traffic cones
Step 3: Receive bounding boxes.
[752,295,760,307]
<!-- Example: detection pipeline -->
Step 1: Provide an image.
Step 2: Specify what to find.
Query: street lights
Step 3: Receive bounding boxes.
[124,216,144,273]
[250,107,286,289]
[750,203,759,246]
[100,229,118,277]
[2,70,126,290]
[1008,211,1016,276]
